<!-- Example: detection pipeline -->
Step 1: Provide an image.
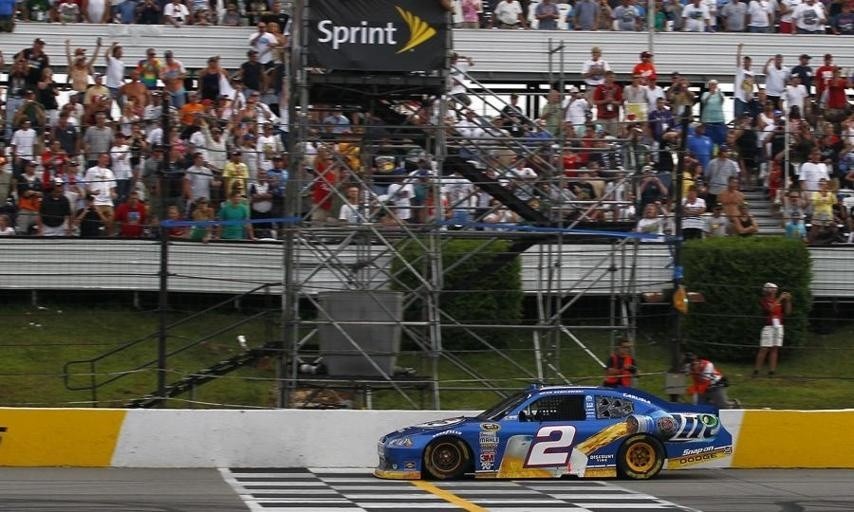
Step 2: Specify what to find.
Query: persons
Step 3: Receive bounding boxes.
[602,331,637,388]
[751,282,792,379]
[683,353,742,410]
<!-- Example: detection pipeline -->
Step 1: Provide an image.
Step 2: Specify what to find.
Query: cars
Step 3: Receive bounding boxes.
[371,382,735,482]
